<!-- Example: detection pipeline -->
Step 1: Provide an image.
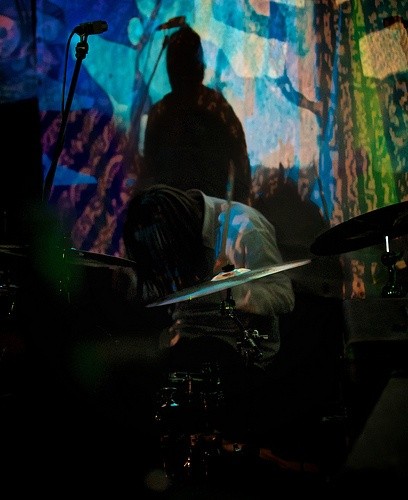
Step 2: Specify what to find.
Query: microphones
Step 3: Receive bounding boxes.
[75,21,107,34]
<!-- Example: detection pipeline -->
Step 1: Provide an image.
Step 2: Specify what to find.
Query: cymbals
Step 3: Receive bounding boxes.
[148,258,312,308]
[0,245,136,267]
[308,200,408,256]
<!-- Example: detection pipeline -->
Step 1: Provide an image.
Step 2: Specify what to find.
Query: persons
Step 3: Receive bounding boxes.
[142,28,253,204]
[95,183,297,400]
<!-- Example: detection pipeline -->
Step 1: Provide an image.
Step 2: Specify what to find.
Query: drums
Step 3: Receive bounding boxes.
[145,369,228,416]
